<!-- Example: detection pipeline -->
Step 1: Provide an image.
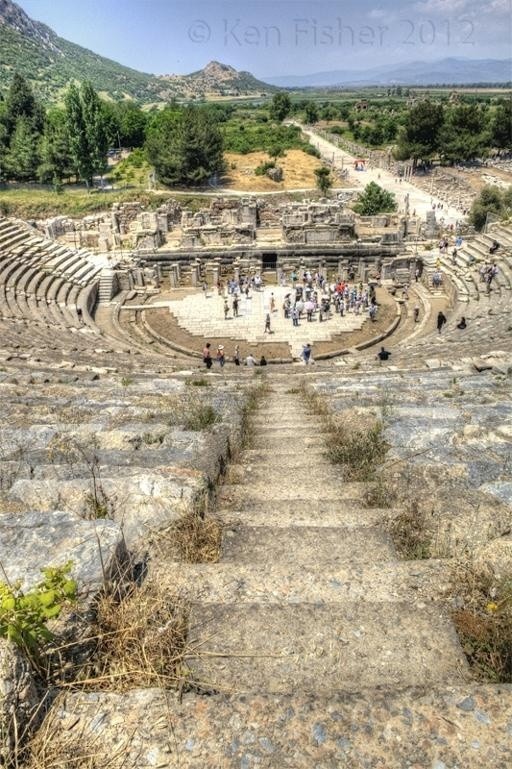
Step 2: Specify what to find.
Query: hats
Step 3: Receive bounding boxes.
[218,345,224,349]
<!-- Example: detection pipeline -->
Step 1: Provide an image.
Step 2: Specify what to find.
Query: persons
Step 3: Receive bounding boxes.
[203,343,267,369]
[479,262,499,284]
[378,347,391,360]
[413,308,419,322]
[457,316,466,329]
[432,201,467,231]
[304,344,311,364]
[439,236,462,265]
[489,240,500,254]
[432,257,443,287]
[437,311,446,334]
[283,265,377,326]
[415,258,424,282]
[203,274,275,333]
